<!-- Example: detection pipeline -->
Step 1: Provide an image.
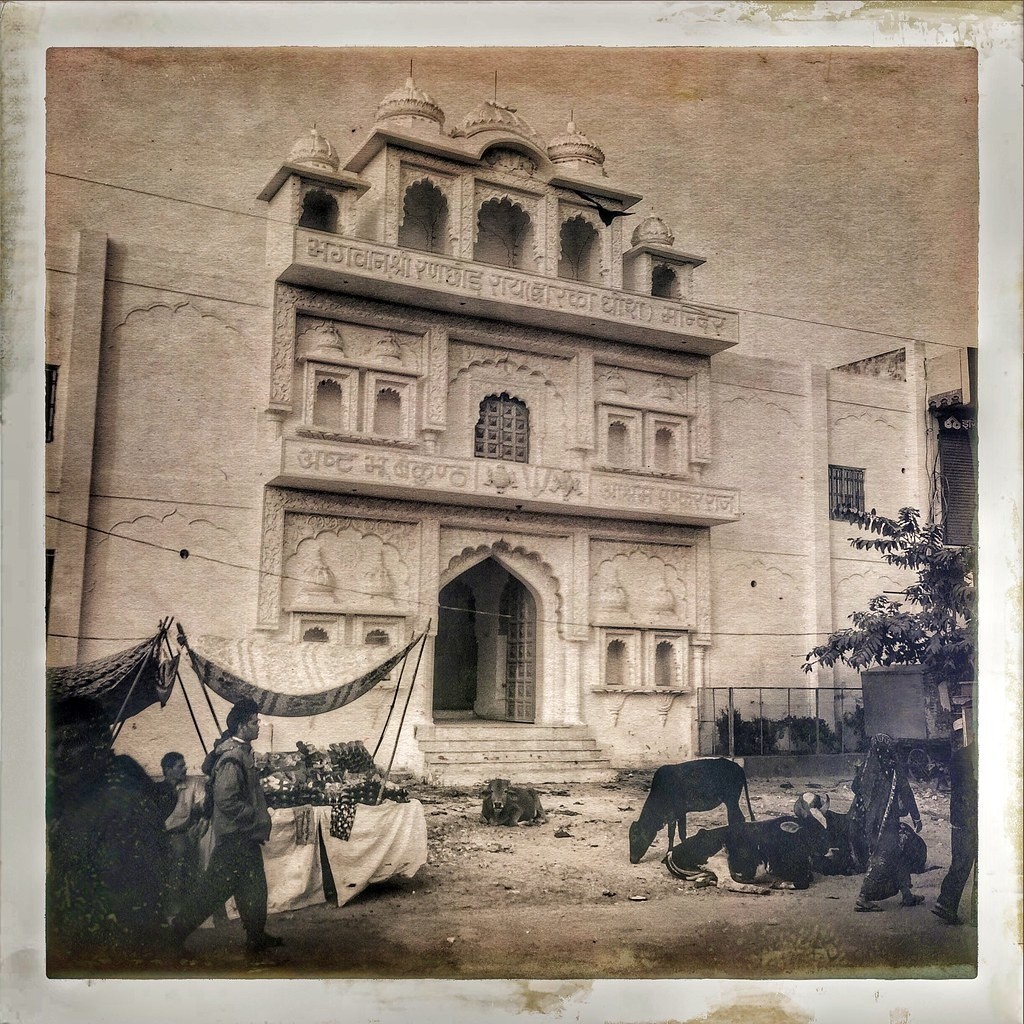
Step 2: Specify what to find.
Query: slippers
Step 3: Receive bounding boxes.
[854,903,883,911]
[900,895,924,906]
[930,906,964,925]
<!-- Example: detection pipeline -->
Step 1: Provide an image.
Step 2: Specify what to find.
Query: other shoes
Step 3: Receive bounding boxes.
[165,925,190,960]
[245,931,284,951]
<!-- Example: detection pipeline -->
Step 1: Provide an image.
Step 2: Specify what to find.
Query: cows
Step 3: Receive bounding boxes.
[479,779,550,827]
[628,754,929,895]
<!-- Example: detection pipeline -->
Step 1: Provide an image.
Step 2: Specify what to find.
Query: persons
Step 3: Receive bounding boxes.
[154,752,203,899]
[849,733,928,912]
[931,733,978,924]
[45,695,171,976]
[150,699,290,966]
